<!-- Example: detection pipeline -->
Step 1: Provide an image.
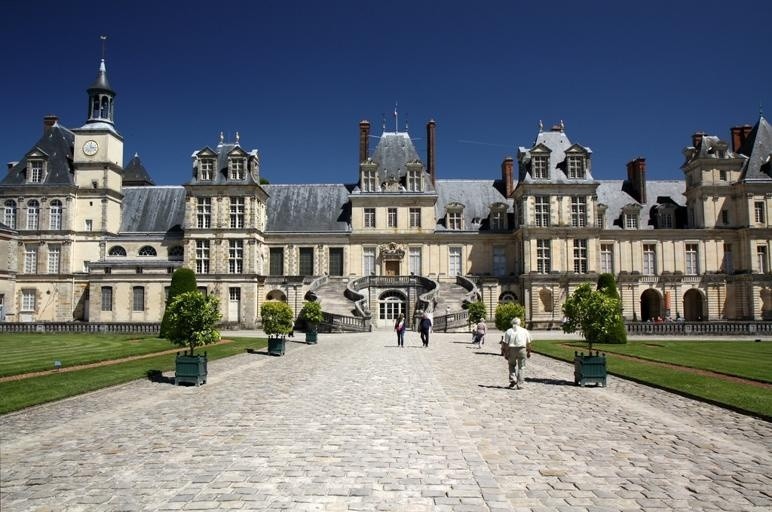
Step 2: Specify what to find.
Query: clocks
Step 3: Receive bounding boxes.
[82,139,99,156]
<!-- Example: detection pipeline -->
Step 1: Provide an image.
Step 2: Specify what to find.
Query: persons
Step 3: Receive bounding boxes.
[503,317,531,390]
[446,306,451,314]
[394,313,406,348]
[647,314,673,323]
[476,317,487,348]
[418,312,432,347]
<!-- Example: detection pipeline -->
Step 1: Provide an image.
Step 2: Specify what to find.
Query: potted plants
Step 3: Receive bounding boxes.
[465,300,488,344]
[160,292,223,387]
[559,282,624,387]
[300,300,323,344]
[261,300,293,355]
[495,302,529,357]
